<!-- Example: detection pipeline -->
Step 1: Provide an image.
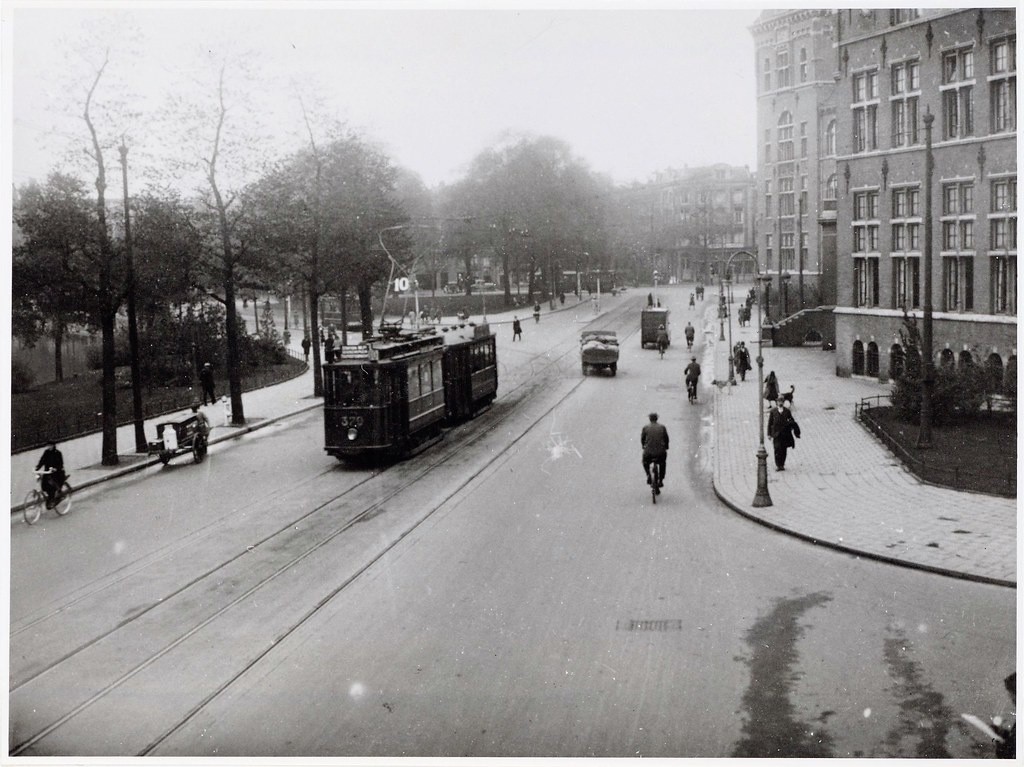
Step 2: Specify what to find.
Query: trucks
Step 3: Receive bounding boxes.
[641,308,670,349]
[581,331,620,377]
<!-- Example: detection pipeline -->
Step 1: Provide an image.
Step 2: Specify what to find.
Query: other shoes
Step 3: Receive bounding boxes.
[686,388,697,400]
[741,375,746,382]
[646,476,666,487]
[47,497,55,509]
[204,399,217,406]
[776,465,785,472]
[737,368,740,374]
[767,405,773,409]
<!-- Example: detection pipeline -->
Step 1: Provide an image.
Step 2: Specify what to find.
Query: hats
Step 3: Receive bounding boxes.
[775,395,786,401]
[648,412,659,421]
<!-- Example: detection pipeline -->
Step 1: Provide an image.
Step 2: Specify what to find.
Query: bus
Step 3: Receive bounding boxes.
[321,327,498,467]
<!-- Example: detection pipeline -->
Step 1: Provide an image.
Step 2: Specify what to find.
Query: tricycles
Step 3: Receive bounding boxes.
[151,412,211,466]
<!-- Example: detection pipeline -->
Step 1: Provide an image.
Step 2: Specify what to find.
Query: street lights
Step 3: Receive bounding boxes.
[721,250,775,507]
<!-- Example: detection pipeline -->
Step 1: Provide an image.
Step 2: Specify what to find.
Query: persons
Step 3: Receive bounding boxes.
[656,323,669,354]
[988,672,1016,760]
[762,370,780,409]
[654,298,661,308]
[32,439,66,509]
[533,301,541,324]
[191,403,211,454]
[688,285,757,328]
[596,302,601,313]
[733,340,752,382]
[560,290,565,308]
[591,297,598,313]
[767,396,795,472]
[408,303,470,330]
[282,309,345,363]
[512,315,523,342]
[640,411,669,488]
[685,322,695,349]
[220,394,233,427]
[684,355,701,399]
[647,292,653,308]
[199,362,218,406]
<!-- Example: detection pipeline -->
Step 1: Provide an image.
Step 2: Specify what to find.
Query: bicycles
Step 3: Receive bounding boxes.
[687,334,694,352]
[658,340,666,359]
[640,444,666,503]
[23,468,74,525]
[684,372,698,404]
